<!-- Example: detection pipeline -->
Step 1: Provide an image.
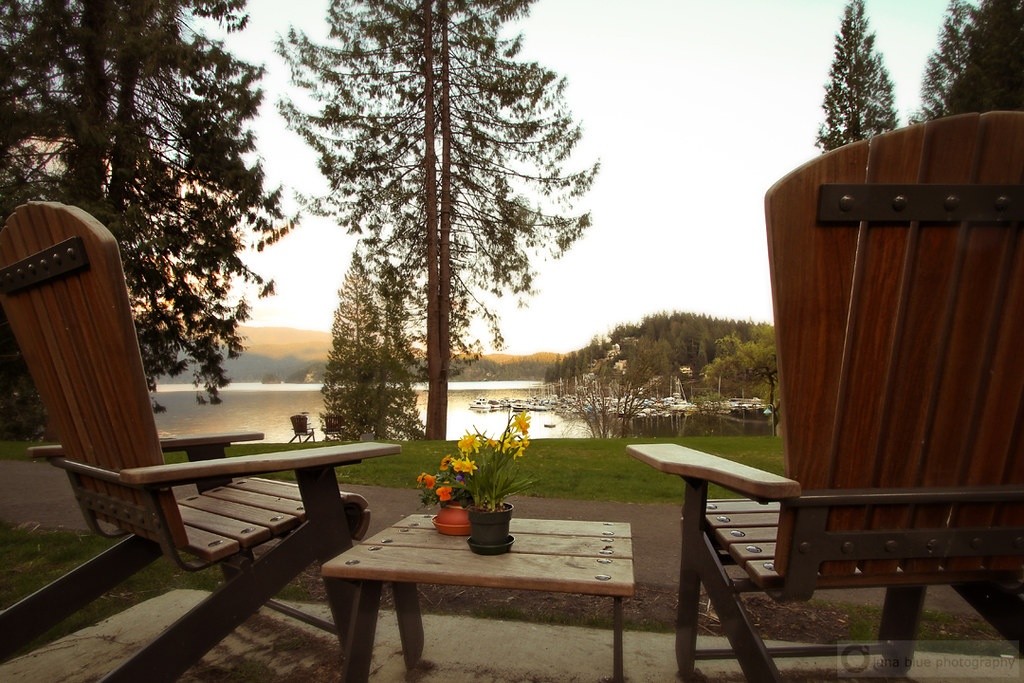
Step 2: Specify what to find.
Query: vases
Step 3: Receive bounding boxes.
[466,503,514,555]
[431,501,472,536]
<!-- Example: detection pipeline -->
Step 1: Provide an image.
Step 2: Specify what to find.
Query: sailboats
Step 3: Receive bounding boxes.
[547,376,698,415]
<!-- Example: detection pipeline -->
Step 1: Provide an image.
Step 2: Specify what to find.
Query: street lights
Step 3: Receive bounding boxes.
[763,404,778,437]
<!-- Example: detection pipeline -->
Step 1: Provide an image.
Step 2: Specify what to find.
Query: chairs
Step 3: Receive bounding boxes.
[323,413,345,442]
[627,105,1024,683]
[288,415,315,444]
[0,200,400,683]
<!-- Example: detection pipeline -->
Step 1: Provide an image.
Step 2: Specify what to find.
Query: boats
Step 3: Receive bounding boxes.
[468,396,553,412]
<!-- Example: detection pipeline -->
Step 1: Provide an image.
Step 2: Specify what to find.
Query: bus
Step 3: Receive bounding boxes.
[728,397,762,406]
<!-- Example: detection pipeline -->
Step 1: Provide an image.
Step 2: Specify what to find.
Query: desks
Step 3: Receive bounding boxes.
[321,512,636,683]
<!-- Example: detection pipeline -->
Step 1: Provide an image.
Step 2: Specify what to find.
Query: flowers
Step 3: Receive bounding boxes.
[434,410,543,511]
[417,410,532,512]
